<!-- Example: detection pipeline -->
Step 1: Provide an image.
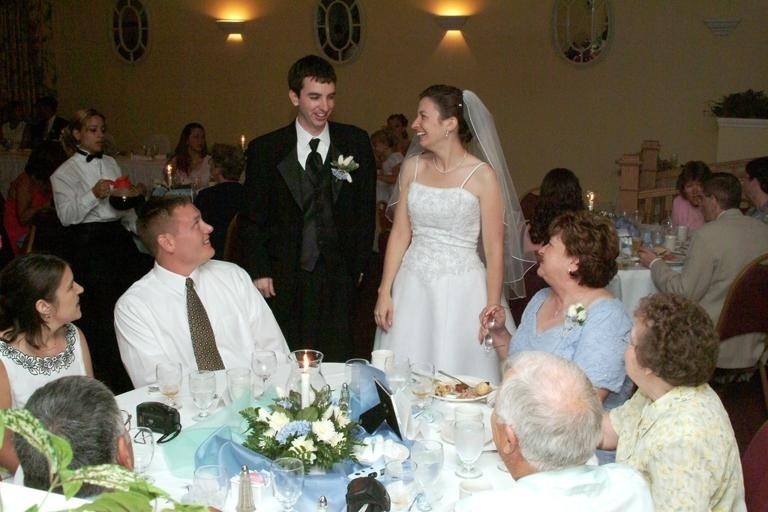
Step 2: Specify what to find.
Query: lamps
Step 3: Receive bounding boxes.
[214,21,248,34]
[431,15,469,31]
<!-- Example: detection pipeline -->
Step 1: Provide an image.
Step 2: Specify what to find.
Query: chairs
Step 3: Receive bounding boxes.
[377,201,393,254]
[506,248,545,326]
[739,199,751,215]
[521,186,543,219]
[30,206,59,231]
[222,211,241,263]
[742,420,767,512]
[711,252,768,404]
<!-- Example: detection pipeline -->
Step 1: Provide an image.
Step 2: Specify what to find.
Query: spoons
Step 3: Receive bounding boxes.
[436,367,472,388]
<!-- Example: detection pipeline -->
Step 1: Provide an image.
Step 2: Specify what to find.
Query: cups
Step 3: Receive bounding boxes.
[344,357,368,382]
[664,234,676,252]
[454,421,483,476]
[155,362,182,408]
[124,427,154,476]
[410,440,444,509]
[406,362,435,415]
[632,236,643,256]
[450,405,484,422]
[250,350,278,401]
[677,225,688,242]
[652,231,663,246]
[188,370,216,423]
[191,464,230,511]
[271,456,304,512]
[372,348,394,373]
[225,367,251,406]
[288,348,323,388]
[385,354,409,399]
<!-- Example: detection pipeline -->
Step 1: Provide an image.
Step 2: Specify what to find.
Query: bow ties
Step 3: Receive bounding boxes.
[75,149,104,162]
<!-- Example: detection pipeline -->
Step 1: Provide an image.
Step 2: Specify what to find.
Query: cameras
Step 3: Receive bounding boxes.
[136,401,182,443]
[346,471,390,512]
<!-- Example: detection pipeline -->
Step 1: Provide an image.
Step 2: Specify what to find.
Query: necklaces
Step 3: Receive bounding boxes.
[430,152,469,174]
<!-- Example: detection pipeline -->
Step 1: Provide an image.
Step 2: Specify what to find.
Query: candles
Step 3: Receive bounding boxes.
[239,134,247,152]
[167,165,175,192]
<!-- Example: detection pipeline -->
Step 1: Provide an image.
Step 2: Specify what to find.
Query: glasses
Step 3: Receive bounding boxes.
[482,309,507,350]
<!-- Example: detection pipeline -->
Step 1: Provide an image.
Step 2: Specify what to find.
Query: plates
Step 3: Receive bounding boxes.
[429,376,496,404]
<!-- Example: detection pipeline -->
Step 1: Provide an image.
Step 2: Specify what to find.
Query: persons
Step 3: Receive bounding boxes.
[193,143,245,261]
[491,350,653,512]
[26,97,63,149]
[672,159,711,231]
[113,194,295,389]
[510,166,583,329]
[599,292,747,512]
[478,208,636,465]
[49,108,148,303]
[3,139,68,260]
[11,376,135,498]
[239,54,376,363]
[370,113,411,157]
[372,128,405,210]
[741,158,768,226]
[0,254,94,480]
[1,101,32,150]
[371,83,516,389]
[639,172,763,385]
[161,123,215,191]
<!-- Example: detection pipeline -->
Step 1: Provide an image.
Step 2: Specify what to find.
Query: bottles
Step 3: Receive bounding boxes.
[235,465,257,511]
[316,495,329,509]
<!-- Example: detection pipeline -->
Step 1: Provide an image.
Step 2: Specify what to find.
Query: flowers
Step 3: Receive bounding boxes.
[707,90,768,119]
[239,384,370,477]
[330,152,357,186]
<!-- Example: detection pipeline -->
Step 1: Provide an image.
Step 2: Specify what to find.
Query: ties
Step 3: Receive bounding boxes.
[185,278,225,374]
[300,138,326,273]
